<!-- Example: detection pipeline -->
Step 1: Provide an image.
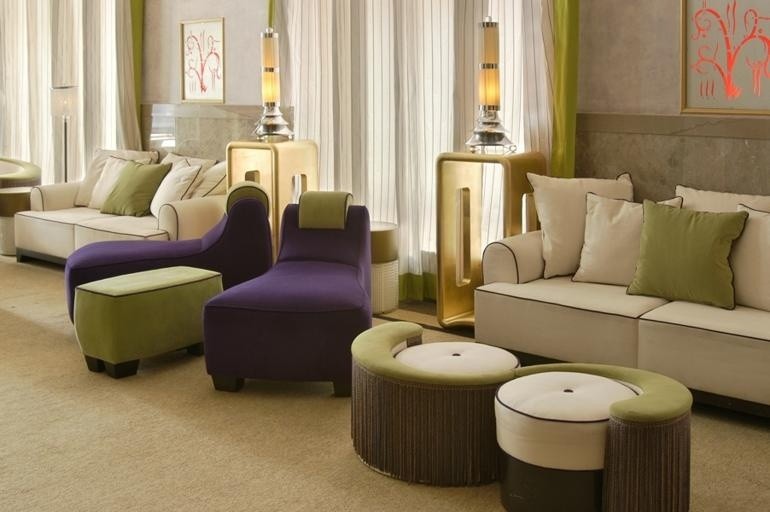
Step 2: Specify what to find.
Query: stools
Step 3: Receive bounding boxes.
[369,220,400,314]
[73,264,223,376]
[1,158,42,256]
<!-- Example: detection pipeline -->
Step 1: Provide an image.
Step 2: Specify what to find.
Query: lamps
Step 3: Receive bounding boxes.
[466,16,516,156]
[49,84,82,181]
[250,28,295,142]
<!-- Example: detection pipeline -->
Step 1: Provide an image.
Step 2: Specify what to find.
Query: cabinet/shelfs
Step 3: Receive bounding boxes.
[435,152,548,331]
[225,139,320,264]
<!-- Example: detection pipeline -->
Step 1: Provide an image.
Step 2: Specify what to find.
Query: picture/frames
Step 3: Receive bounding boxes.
[679,0,770,115]
[179,17,225,104]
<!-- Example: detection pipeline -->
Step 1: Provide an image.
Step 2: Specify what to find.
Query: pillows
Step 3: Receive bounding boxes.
[674,185,768,214]
[525,170,635,279]
[728,203,770,313]
[571,191,683,287]
[73,148,227,217]
[626,198,749,310]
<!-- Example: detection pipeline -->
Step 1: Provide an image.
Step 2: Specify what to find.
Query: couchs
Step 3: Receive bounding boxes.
[202,190,372,398]
[63,181,273,324]
[474,230,770,420]
[13,181,226,267]
